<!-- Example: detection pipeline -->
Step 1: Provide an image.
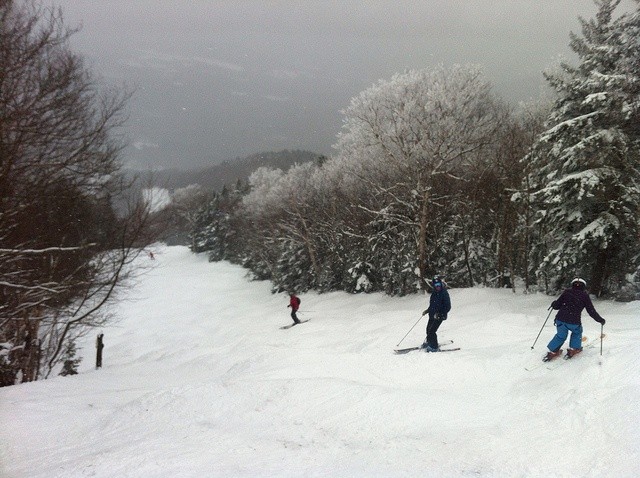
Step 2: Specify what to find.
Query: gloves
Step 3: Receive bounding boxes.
[422,310,428,316]
[600,319,605,325]
[434,313,440,319]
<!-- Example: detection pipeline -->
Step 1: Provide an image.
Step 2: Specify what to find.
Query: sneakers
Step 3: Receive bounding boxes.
[427,346,438,352]
[422,343,429,348]
[550,351,560,356]
[570,347,581,354]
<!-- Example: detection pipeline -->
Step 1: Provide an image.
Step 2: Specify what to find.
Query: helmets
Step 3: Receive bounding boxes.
[571,278,587,286]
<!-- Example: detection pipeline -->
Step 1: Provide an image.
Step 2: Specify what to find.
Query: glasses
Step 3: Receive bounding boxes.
[434,283,441,287]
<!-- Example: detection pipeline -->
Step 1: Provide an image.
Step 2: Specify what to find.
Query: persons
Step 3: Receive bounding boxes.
[547,277,606,357]
[420,274,452,352]
[287,290,301,325]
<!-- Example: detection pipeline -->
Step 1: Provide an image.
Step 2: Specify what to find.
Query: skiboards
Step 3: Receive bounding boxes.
[394,340,461,353]
[281,318,310,330]
[523,334,608,371]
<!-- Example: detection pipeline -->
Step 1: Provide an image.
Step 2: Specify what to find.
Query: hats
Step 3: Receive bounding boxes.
[432,278,442,287]
